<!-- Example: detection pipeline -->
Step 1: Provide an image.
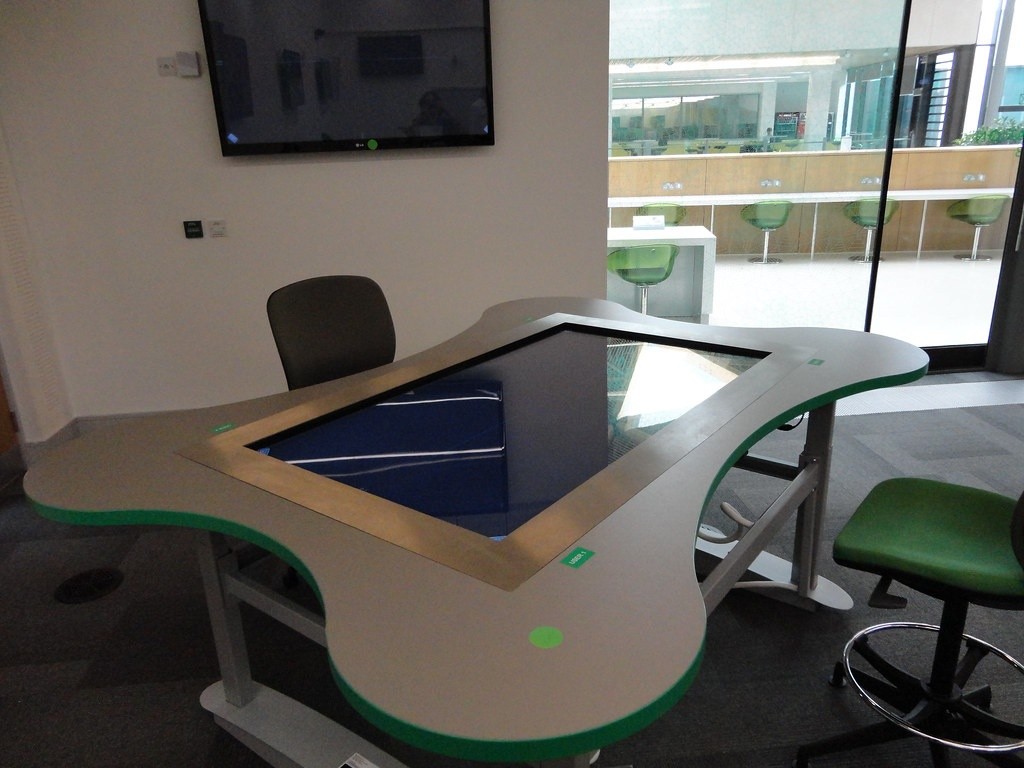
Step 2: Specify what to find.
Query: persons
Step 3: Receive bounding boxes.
[762,127,773,152]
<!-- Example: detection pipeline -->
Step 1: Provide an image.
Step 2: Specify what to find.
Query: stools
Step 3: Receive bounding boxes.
[606,244,680,316]
[842,195,900,263]
[635,202,687,226]
[946,195,1010,261]
[740,200,794,264]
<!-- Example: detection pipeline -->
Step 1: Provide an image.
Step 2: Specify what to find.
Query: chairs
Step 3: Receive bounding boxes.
[794,477,1024,768]
[266,274,396,391]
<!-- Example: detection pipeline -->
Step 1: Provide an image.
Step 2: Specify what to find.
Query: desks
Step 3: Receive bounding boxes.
[606,226,717,326]
[21,295,932,768]
[608,187,1015,261]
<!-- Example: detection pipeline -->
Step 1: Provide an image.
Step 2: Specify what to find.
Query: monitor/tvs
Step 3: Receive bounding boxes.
[197,0,496,159]
[249,381,510,519]
